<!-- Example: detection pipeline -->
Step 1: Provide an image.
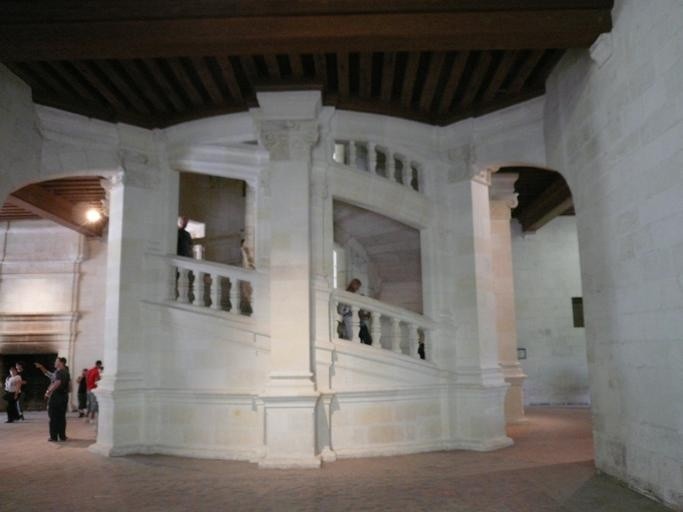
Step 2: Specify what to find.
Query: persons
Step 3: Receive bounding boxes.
[3,356,105,440]
[176,214,193,258]
[341,278,361,341]
